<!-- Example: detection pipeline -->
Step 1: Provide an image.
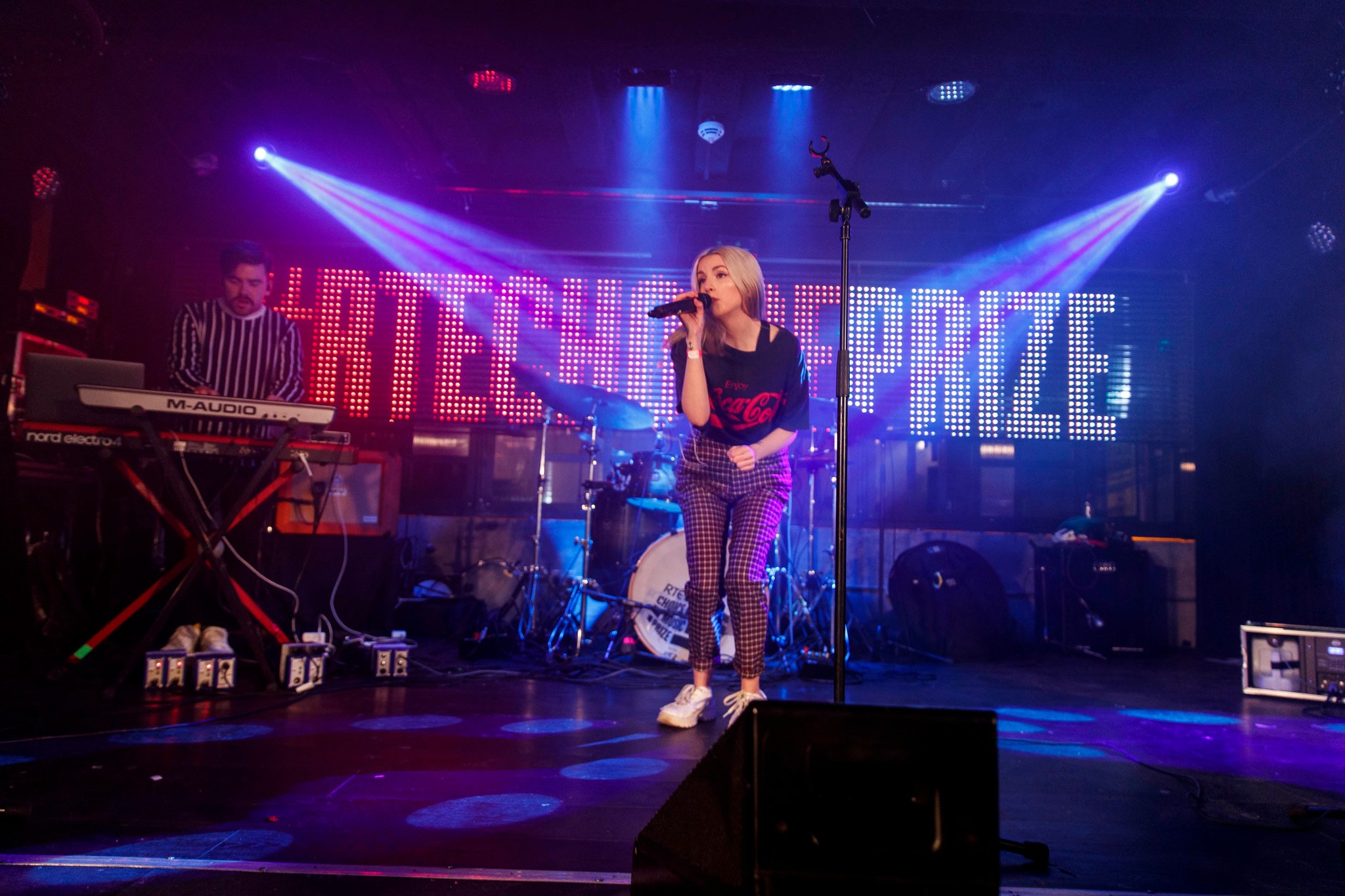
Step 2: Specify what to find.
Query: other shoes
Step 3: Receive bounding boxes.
[160,624,199,655]
[200,625,233,652]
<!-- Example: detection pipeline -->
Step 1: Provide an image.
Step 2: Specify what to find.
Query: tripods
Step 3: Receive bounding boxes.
[471,403,641,670]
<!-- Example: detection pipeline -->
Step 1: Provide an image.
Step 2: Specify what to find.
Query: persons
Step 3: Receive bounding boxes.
[159,242,305,652]
[656,246,808,727]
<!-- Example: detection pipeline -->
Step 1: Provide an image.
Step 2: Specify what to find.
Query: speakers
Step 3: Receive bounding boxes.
[629,697,1002,896]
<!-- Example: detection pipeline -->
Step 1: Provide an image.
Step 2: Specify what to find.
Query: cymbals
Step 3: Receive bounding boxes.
[797,456,835,468]
[807,395,861,426]
[535,379,656,431]
[510,364,555,405]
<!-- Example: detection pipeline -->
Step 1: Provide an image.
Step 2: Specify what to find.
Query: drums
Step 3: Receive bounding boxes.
[586,489,680,602]
[626,527,770,665]
[623,451,682,514]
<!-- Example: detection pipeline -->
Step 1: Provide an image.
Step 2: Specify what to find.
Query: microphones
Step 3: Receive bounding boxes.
[1002,839,1050,863]
[646,292,711,322]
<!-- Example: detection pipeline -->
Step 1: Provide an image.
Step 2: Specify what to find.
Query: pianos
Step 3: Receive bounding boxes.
[18,380,363,666]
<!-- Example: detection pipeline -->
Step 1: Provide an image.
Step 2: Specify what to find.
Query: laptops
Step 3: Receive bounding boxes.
[23,353,144,429]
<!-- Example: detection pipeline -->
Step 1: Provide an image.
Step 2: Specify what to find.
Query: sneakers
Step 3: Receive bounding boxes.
[722,688,767,727]
[655,681,712,727]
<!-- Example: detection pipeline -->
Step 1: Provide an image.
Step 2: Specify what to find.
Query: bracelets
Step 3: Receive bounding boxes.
[686,349,702,359]
[748,445,758,462]
